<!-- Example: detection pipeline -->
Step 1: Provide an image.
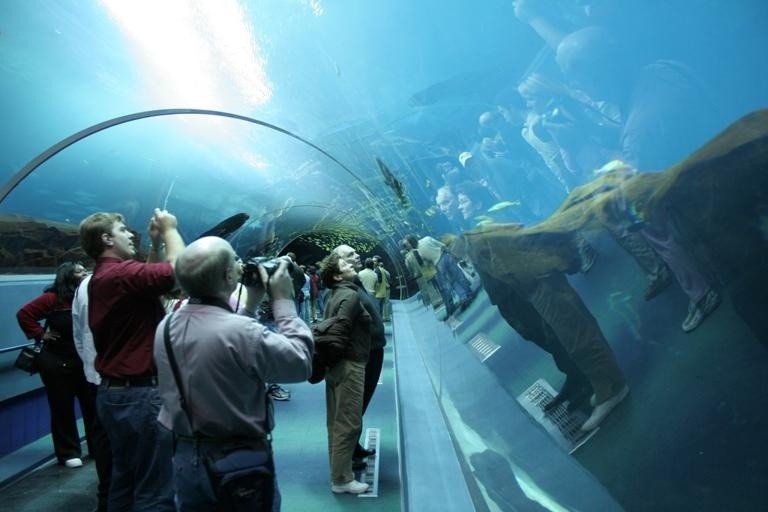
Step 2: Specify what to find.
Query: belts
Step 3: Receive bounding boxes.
[101,375,158,387]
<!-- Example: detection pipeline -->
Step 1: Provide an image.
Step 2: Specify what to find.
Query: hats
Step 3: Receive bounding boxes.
[459,152,473,169]
[594,161,630,176]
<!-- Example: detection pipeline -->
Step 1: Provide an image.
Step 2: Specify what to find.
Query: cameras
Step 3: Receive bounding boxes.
[532,103,578,141]
[243,257,291,288]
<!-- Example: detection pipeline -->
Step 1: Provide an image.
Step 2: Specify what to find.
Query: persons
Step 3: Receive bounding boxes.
[14,205,392,512]
[396,2,768,436]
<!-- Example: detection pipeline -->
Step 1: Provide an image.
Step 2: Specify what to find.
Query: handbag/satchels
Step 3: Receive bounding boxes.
[15,343,42,375]
[592,122,623,160]
[205,439,275,510]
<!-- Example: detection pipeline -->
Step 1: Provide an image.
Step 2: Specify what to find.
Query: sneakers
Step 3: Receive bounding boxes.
[642,270,673,301]
[331,479,370,494]
[65,457,83,468]
[310,317,318,323]
[581,386,630,431]
[579,247,598,273]
[268,385,291,400]
[682,289,722,333]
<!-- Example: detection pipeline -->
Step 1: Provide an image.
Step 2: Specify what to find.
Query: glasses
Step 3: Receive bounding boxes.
[230,254,243,271]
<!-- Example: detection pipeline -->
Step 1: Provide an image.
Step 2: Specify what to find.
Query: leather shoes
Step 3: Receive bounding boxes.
[351,460,367,468]
[544,381,592,411]
[353,448,375,459]
